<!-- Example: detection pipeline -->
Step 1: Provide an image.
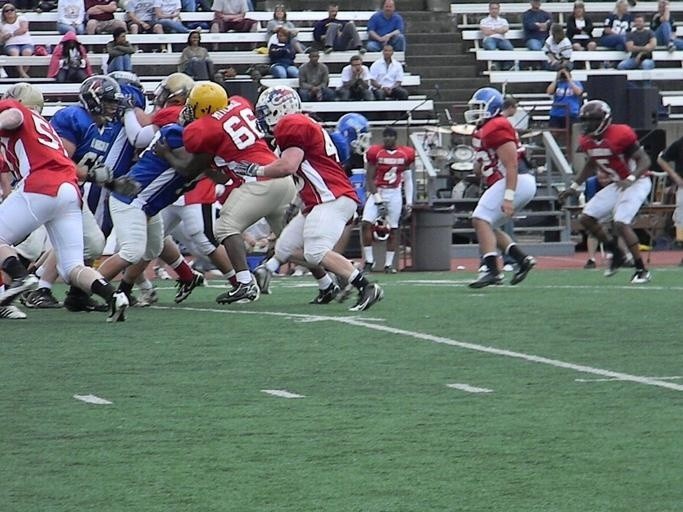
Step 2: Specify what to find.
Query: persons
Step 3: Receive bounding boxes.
[652,2,683,51]
[596,17,627,52]
[156,2,192,34]
[57,0,85,34]
[581,167,635,269]
[25,74,125,307]
[657,135,683,255]
[19,72,144,307]
[0,83,127,321]
[2,2,35,77]
[524,2,552,50]
[617,17,659,68]
[467,88,537,286]
[537,67,588,174]
[66,78,228,312]
[48,34,92,82]
[369,44,410,101]
[497,98,517,117]
[268,4,301,53]
[481,2,516,70]
[328,113,370,175]
[359,130,417,272]
[105,26,133,73]
[270,28,299,77]
[313,4,364,54]
[153,76,340,306]
[234,86,383,312]
[142,71,244,305]
[568,3,597,50]
[340,56,374,100]
[84,0,127,37]
[297,49,336,98]
[211,0,254,33]
[608,1,635,26]
[542,26,574,69]
[119,0,162,35]
[574,101,651,283]
[368,0,405,51]
[183,31,213,80]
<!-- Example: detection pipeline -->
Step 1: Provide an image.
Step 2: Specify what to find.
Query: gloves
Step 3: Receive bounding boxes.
[87,162,113,182]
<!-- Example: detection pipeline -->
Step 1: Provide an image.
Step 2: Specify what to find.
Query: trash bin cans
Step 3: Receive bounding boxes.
[410,205,454,271]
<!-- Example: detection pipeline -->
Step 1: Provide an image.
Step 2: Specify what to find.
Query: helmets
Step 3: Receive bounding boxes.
[255,86,302,133]
[79,75,125,121]
[464,87,505,124]
[154,72,195,106]
[579,100,613,138]
[4,83,44,114]
[108,70,147,111]
[337,113,372,156]
[188,81,229,119]
[372,218,391,241]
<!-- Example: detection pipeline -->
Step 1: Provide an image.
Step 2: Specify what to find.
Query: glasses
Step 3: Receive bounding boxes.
[4,8,15,12]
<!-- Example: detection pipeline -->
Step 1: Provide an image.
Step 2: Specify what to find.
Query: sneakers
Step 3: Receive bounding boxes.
[171,269,205,303]
[309,282,339,305]
[383,264,397,274]
[106,290,129,323]
[603,253,630,277]
[336,278,357,302]
[130,294,138,306]
[348,280,384,311]
[254,264,272,295]
[510,255,537,285]
[629,269,652,283]
[140,288,158,307]
[1,305,27,319]
[468,264,504,288]
[215,276,260,304]
[0,275,39,306]
[28,288,64,308]
[292,268,304,276]
[65,292,109,312]
[363,260,376,274]
[584,259,596,268]
